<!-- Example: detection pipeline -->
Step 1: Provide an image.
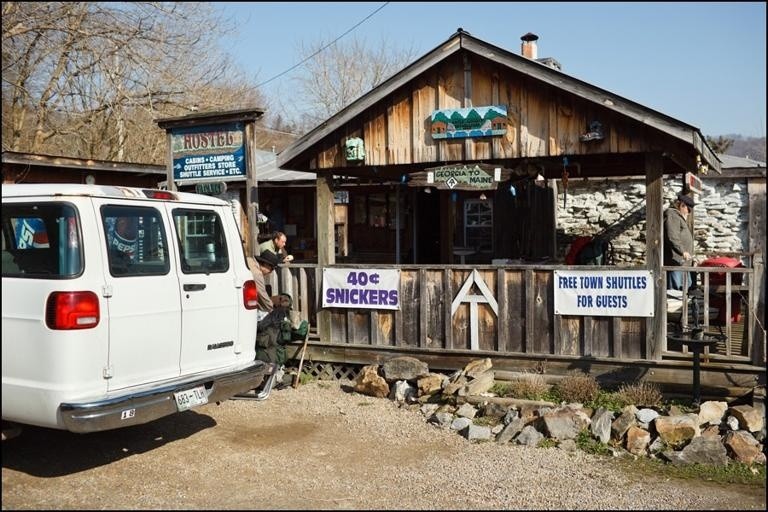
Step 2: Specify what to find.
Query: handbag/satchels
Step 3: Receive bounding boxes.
[565,236,595,265]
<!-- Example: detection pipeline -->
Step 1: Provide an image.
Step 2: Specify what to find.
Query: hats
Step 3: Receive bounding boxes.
[678,194,695,208]
[255,249,281,270]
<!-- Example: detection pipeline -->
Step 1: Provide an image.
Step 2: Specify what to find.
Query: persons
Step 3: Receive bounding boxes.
[251,229,295,301]
[253,288,314,393]
[660,196,697,306]
[243,249,280,313]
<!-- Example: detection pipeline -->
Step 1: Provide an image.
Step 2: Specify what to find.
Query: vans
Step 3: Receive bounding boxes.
[0,178,270,439]
